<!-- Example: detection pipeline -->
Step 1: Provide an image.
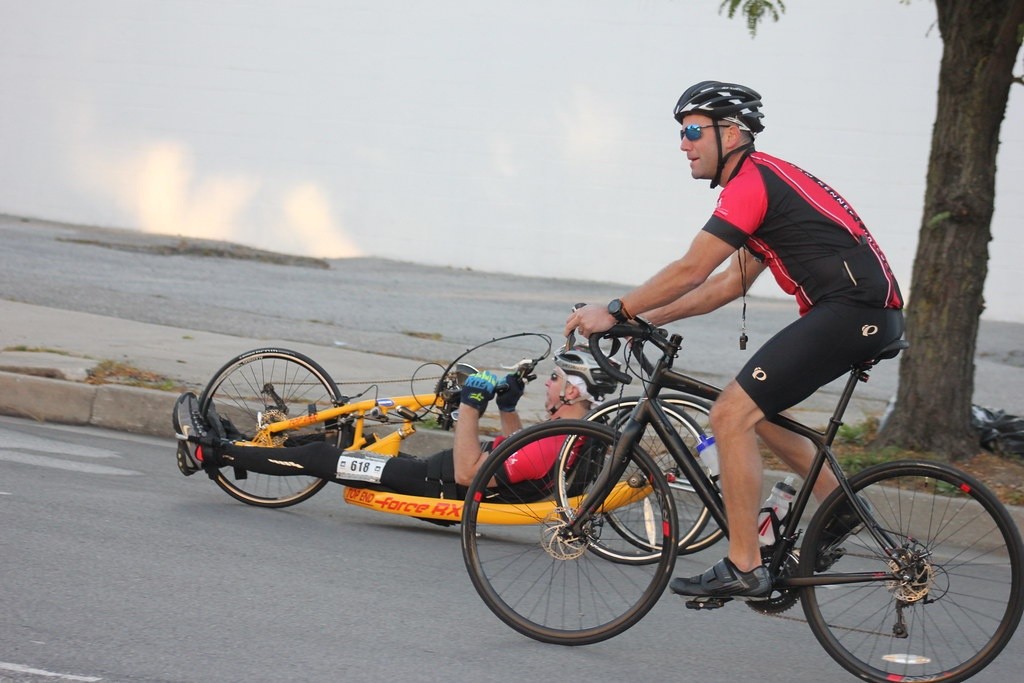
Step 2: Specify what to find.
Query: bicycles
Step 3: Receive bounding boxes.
[461,304,1024,683]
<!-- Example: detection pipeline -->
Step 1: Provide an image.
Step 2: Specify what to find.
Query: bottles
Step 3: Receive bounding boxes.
[757,475,797,545]
[697,433,724,498]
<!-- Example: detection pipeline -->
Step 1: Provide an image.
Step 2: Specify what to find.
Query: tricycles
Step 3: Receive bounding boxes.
[177,347,736,566]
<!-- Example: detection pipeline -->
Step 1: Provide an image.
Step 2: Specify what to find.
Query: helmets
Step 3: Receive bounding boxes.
[551,341,622,409]
[673,80,765,140]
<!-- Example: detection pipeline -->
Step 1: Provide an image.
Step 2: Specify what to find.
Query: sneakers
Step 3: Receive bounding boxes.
[813,495,872,559]
[670,557,772,601]
[172,392,230,477]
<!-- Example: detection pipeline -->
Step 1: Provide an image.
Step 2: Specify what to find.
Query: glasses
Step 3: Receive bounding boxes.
[550,373,559,382]
[679,124,731,142]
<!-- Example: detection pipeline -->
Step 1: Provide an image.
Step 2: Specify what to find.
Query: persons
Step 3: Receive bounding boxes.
[563,81,905,596]
[171,342,623,505]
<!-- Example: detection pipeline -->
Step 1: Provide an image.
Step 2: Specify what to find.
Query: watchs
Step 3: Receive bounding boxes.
[606,298,631,323]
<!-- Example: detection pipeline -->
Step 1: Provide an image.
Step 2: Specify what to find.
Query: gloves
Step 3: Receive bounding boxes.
[461,369,498,418]
[496,374,525,412]
